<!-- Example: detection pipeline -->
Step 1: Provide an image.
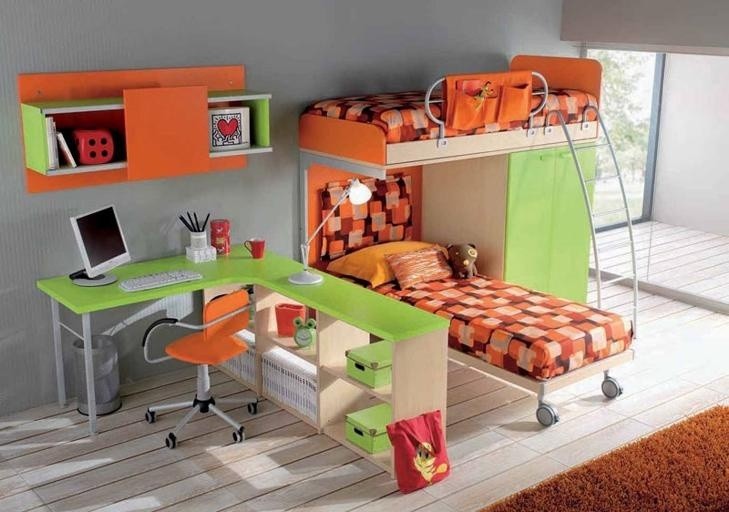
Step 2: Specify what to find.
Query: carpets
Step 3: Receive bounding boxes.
[476,402,729,511]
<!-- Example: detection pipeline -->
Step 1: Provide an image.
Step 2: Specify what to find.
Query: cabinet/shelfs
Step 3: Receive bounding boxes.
[21,88,275,177]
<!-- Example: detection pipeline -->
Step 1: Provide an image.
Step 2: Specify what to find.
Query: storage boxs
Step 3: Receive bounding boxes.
[344,337,393,391]
[344,403,396,456]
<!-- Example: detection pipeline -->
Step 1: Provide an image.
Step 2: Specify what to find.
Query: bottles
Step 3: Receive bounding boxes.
[211,220,230,255]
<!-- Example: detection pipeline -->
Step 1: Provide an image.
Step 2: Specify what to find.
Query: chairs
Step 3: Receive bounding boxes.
[141,284,260,452]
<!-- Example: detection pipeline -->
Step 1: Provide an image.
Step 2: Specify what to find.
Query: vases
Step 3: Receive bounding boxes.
[274,301,308,339]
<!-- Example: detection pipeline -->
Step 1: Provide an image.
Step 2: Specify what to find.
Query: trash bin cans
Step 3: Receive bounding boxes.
[72,335,123,417]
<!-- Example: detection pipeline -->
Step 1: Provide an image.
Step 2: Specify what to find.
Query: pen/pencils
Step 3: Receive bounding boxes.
[178,211,210,232]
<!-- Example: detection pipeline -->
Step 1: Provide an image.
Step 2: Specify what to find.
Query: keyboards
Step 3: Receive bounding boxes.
[119,270,202,293]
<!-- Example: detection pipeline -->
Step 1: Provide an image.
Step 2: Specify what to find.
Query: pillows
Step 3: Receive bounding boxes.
[326,236,436,291]
[383,244,454,291]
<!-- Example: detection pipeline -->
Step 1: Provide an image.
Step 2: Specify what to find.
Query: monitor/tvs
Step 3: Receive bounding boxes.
[69,204,131,286]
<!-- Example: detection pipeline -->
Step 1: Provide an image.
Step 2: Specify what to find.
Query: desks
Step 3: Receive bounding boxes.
[34,243,451,483]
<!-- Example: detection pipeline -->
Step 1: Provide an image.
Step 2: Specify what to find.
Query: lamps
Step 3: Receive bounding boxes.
[286,177,372,286]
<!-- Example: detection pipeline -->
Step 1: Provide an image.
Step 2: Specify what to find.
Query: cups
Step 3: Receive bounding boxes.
[190,229,207,250]
[242,237,265,259]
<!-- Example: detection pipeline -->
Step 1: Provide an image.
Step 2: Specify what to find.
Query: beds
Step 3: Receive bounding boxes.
[297,52,641,427]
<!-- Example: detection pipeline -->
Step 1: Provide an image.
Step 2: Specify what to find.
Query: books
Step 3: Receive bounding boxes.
[221,329,317,424]
